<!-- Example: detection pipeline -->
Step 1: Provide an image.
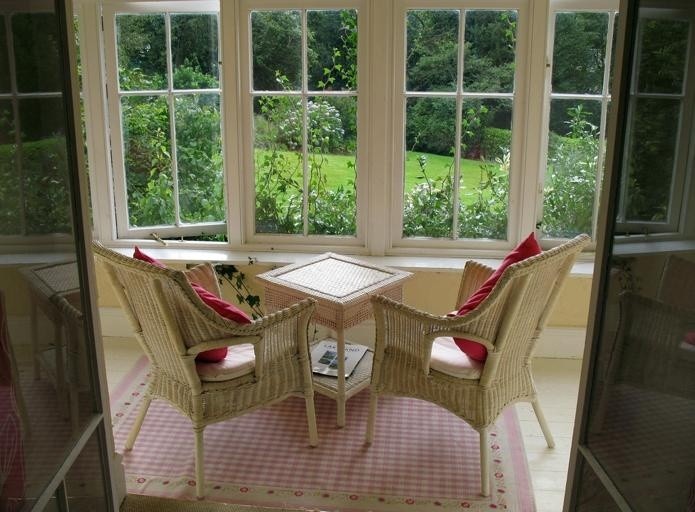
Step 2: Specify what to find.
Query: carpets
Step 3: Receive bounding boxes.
[579,338,694,509]
[15,363,109,487]
[110,336,536,512]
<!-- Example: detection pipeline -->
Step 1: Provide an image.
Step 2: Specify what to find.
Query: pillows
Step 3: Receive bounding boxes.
[683,329,693,343]
[133,245,252,363]
[448,232,543,363]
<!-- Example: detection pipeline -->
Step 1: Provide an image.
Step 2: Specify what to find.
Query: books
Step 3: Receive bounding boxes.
[312,340,369,380]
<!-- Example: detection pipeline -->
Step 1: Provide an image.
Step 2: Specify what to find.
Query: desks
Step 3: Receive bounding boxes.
[252,252,416,428]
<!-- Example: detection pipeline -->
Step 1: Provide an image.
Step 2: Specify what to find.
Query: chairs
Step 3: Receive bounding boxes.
[594,254,694,434]
[366,234,591,498]
[91,238,319,501]
[54,288,91,453]
[0,282,33,441]
